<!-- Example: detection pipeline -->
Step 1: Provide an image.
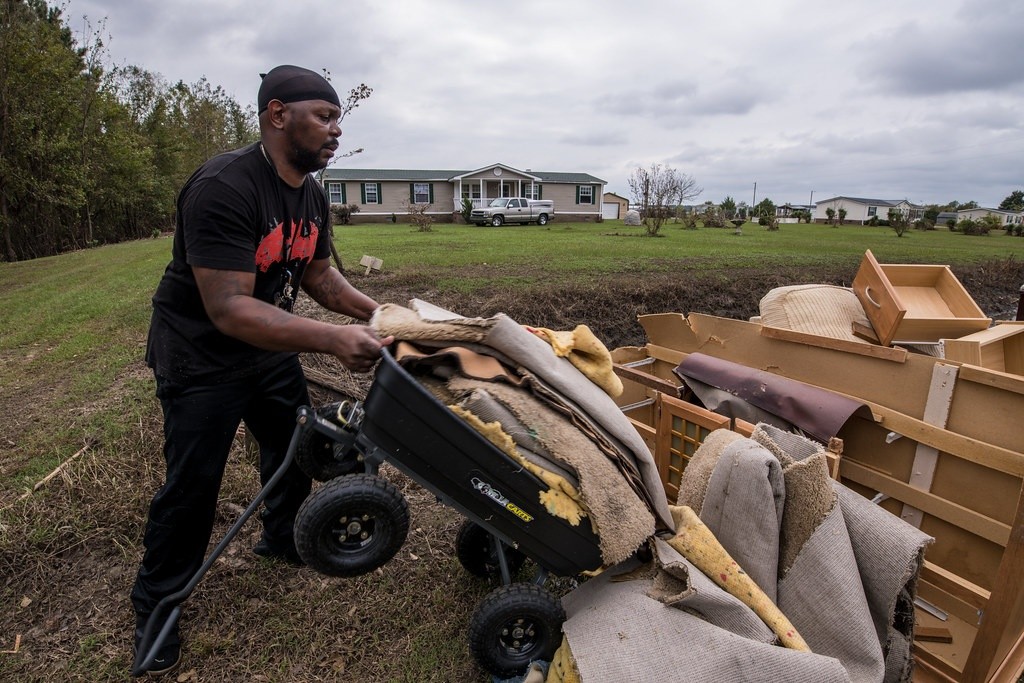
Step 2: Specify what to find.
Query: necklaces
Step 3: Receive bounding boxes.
[261,143,277,177]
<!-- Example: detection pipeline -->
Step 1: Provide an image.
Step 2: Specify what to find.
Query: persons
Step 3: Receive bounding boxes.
[129,65,394,675]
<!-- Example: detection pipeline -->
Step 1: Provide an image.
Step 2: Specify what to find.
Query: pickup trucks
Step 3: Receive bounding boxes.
[469,197,555,227]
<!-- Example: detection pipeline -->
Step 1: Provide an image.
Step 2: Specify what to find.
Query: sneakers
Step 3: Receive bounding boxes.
[252,544,304,566]
[134,612,182,677]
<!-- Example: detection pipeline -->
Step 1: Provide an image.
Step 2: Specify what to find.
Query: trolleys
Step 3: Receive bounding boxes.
[128,345,653,680]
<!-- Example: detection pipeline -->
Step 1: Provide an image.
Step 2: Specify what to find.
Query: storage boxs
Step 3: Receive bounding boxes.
[945,320,1024,377]
[850,249,992,346]
